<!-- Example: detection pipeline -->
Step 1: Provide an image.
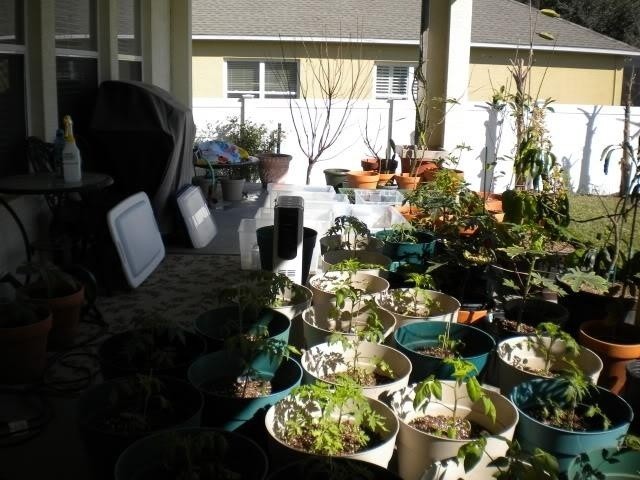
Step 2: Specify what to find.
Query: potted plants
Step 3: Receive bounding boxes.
[0,258,84,352]
[0,303,52,386]
[102,0,640,480]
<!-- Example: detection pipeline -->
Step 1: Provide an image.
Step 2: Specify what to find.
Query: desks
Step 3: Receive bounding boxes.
[0,172,114,329]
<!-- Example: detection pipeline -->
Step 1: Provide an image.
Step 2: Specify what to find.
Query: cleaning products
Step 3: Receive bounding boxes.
[61,115,82,184]
